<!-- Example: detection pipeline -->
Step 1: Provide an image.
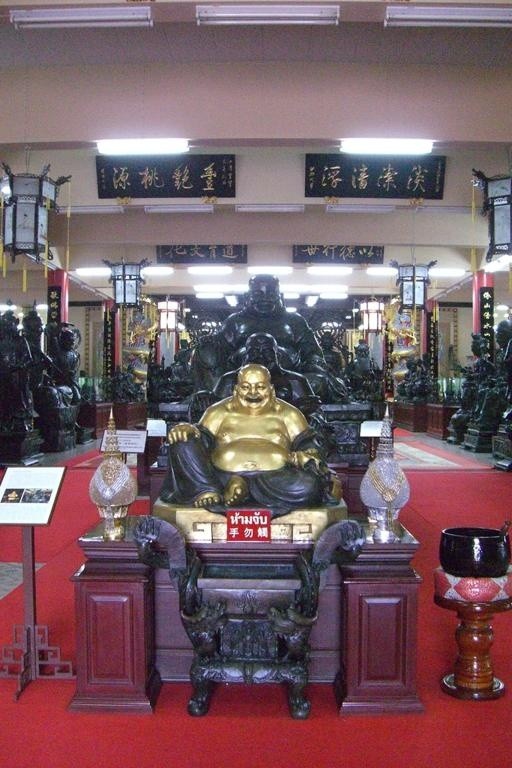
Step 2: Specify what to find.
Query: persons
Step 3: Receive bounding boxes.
[20,307,47,390]
[157,363,344,511]
[483,318,512,419]
[0,310,38,432]
[318,332,342,374]
[182,273,350,402]
[413,359,424,381]
[50,319,85,404]
[467,333,494,400]
[355,339,369,371]
[188,331,323,432]
[458,365,473,412]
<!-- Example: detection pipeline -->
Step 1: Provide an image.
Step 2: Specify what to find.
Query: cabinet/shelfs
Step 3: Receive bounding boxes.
[80,400,148,439]
[68,514,430,719]
[395,395,463,441]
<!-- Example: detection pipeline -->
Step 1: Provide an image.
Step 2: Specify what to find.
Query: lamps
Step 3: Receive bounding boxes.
[390,258,440,316]
[357,297,388,337]
[157,299,181,334]
[0,159,71,264]
[471,167,512,262]
[104,255,150,311]
